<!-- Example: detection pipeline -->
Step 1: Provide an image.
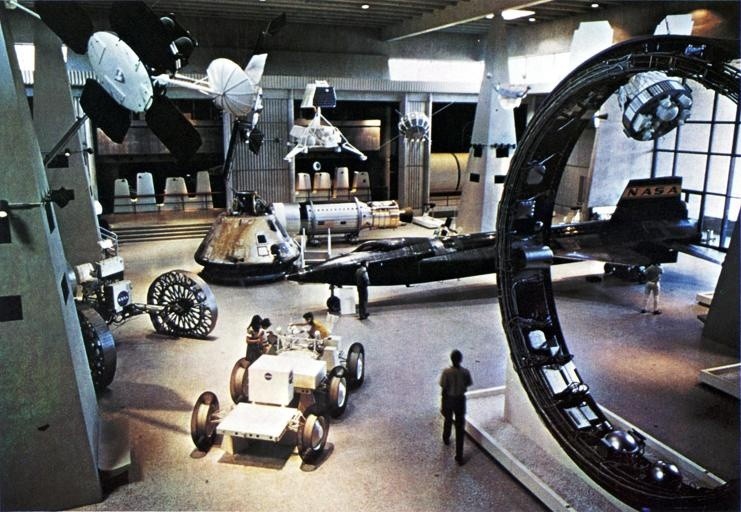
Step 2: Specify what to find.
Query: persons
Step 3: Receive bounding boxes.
[438,348,474,467]
[640,264,664,315]
[262,318,272,333]
[353,260,371,320]
[288,311,330,339]
[245,314,269,363]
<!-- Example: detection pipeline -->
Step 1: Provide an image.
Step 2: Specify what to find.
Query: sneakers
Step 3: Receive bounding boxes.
[443,434,450,446]
[359,312,369,320]
[454,455,464,467]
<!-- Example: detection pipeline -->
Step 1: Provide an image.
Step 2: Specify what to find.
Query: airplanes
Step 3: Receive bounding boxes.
[288,178,702,314]
